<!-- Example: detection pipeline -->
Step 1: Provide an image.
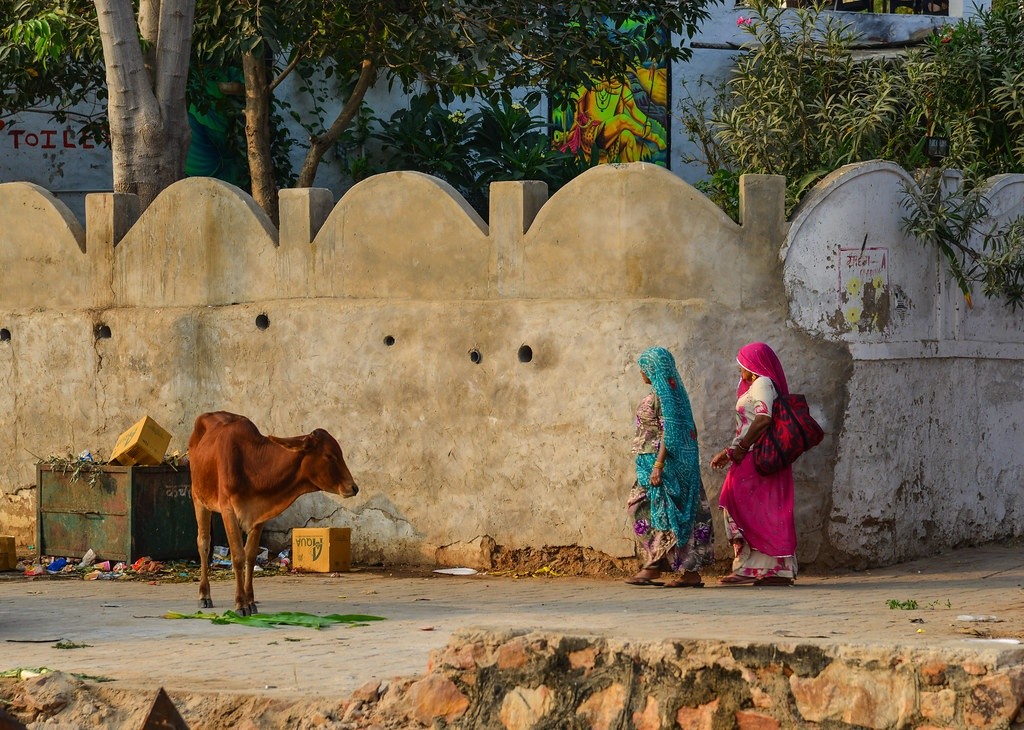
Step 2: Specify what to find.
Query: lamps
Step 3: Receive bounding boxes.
[923,137,950,163]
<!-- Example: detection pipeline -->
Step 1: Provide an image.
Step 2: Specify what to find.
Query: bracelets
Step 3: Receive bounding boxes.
[738,442,748,451]
[654,462,664,468]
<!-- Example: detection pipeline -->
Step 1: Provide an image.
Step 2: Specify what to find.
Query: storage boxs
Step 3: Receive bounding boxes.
[0,535,17,572]
[108,415,172,466]
[293,526,352,571]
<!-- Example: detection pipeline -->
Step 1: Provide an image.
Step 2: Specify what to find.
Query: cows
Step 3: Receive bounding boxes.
[187,411,359,617]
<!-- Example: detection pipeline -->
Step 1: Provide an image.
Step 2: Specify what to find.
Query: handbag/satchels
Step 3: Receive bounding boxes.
[752,378,824,477]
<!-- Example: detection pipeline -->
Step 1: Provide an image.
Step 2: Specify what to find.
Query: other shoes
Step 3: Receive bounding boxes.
[717,574,757,584]
[753,577,791,586]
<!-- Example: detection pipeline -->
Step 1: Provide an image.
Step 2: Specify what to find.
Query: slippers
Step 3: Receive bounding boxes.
[663,580,705,588]
[625,577,665,586]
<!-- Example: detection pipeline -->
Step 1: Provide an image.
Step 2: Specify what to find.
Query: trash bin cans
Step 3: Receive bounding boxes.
[34,464,228,566]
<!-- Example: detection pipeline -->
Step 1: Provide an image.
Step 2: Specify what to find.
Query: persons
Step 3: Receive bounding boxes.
[710,342,798,588]
[625,348,716,588]
